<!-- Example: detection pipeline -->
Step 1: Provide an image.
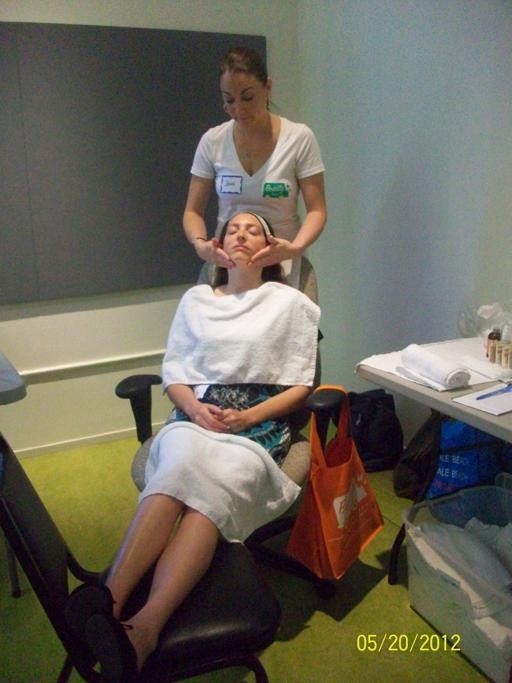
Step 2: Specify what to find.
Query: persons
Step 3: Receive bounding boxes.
[179,45,330,269]
[62,211,323,683]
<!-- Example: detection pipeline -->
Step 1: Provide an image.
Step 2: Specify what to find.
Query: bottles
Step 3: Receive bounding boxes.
[486,321,512,370]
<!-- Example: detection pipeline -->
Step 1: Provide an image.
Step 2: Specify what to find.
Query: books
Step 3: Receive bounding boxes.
[450,382,512,414]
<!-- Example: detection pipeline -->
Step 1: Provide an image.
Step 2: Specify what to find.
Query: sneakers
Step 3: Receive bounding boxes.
[62,581,141,683]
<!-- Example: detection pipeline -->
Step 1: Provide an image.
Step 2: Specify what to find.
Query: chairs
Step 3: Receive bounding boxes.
[115,374,348,582]
[1,429,281,678]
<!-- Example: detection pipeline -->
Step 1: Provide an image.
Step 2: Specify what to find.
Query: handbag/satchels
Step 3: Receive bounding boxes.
[348,388,404,472]
[395,408,505,502]
[286,384,384,583]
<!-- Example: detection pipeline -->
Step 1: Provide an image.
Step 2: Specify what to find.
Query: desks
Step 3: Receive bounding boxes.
[356,336,511,586]
[1,350,27,599]
